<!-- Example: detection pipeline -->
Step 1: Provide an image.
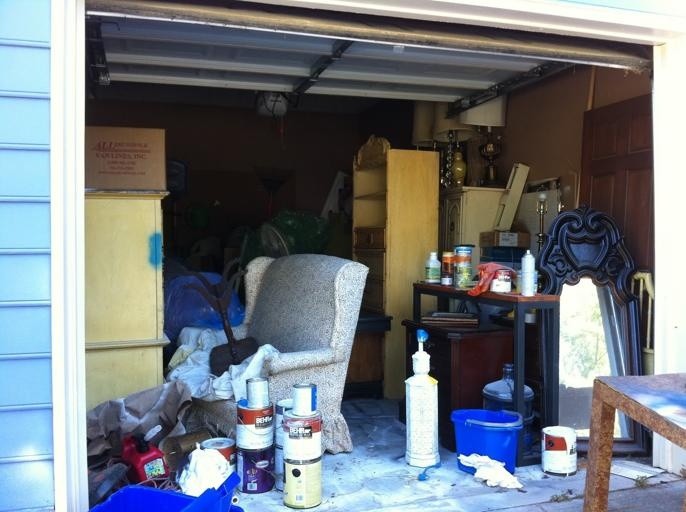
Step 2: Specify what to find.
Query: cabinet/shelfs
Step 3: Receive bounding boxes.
[350,147,440,399]
[444,186,505,261]
[410,281,558,468]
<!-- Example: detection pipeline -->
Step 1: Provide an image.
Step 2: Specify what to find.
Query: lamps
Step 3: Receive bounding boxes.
[432,103,476,187]
[460,96,510,186]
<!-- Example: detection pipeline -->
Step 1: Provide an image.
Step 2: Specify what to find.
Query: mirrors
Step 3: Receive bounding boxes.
[538,204,647,457]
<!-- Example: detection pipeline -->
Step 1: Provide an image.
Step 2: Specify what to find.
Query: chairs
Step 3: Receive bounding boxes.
[178,255,367,454]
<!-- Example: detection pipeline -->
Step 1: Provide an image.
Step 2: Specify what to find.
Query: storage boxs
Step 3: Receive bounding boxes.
[85,127,165,190]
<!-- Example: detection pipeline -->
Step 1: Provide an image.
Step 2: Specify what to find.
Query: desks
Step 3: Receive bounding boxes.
[579,371,685,511]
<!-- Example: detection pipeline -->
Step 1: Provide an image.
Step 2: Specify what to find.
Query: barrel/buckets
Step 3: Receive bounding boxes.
[515,270,538,294]
[454,246,472,267]
[442,251,454,275]
[283,458,323,510]
[451,407,524,478]
[235,445,276,494]
[541,425,577,477]
[481,362,535,455]
[236,399,274,450]
[273,445,283,491]
[201,436,236,473]
[274,399,292,447]
[490,269,512,293]
[454,267,472,289]
[283,411,323,462]
[293,383,317,416]
[246,377,269,408]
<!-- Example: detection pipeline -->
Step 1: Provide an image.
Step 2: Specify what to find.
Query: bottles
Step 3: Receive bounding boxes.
[521,249,536,297]
